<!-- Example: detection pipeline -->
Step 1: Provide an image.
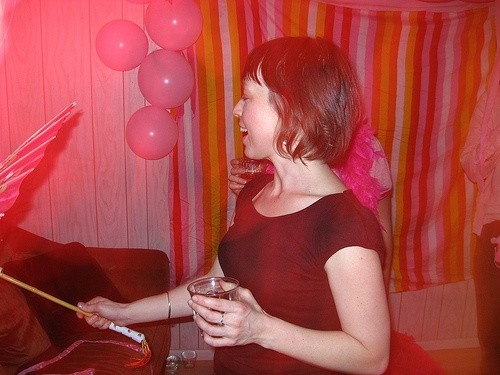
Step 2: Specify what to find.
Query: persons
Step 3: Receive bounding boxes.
[458,15,500,375]
[230,121,392,295]
[78,34,392,375]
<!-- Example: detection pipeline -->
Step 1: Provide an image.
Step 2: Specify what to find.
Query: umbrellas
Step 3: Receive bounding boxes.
[0,100,151,365]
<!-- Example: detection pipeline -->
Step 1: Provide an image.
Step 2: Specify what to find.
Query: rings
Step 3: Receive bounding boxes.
[220,314,224,325]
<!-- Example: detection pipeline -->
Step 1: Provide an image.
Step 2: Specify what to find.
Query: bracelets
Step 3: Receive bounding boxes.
[167,291,171,319]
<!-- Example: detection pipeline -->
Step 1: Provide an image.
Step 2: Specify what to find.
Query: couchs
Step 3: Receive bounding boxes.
[0,223,172,375]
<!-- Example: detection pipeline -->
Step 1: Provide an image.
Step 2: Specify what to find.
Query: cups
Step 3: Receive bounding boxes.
[181,351,197,368]
[494,246,500,268]
[165,355,181,375]
[238,157,267,184]
[187,277,239,338]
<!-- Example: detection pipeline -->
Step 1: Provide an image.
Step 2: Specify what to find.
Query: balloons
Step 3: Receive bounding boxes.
[138,49,194,108]
[145,0,202,50]
[95,20,148,71]
[126,105,177,160]
